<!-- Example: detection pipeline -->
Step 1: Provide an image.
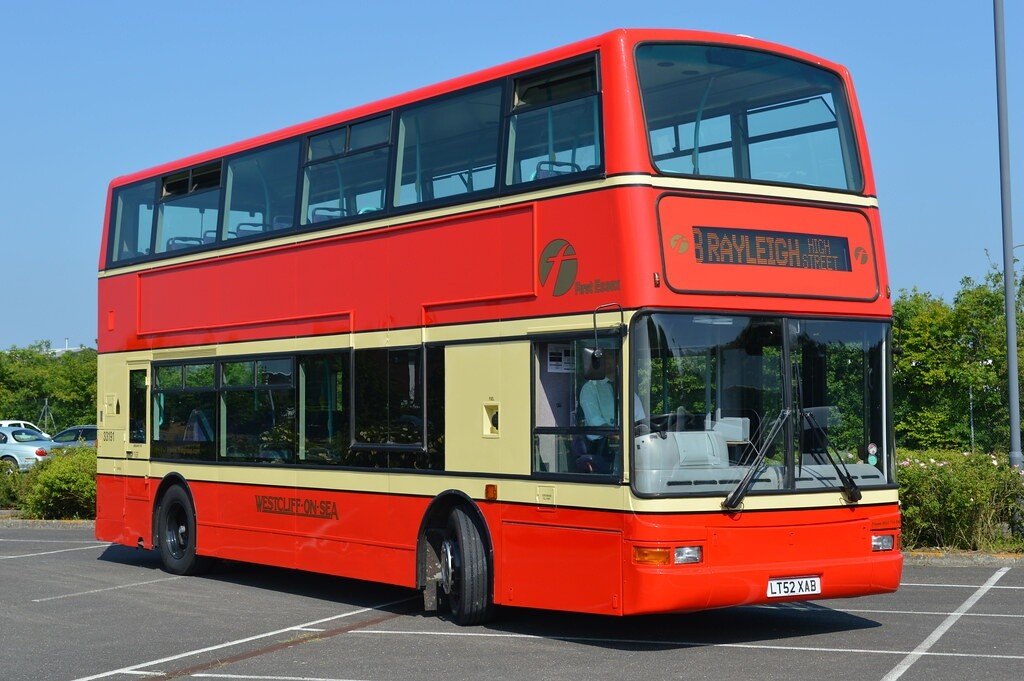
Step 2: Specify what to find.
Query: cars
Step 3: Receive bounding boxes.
[0,426,62,474]
[0,420,51,438]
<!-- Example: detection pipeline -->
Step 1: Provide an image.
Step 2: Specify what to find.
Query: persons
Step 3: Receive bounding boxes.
[579,347,650,473]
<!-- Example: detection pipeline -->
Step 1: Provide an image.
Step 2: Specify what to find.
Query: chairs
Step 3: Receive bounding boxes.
[204,230,236,245]
[536,160,582,182]
[273,216,293,230]
[167,237,204,251]
[312,207,349,225]
[237,223,271,237]
[572,409,614,474]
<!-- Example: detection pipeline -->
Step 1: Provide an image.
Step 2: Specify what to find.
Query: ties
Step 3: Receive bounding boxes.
[607,381,615,392]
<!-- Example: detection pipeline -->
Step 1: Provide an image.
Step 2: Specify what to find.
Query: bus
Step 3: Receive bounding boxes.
[92,24,904,623]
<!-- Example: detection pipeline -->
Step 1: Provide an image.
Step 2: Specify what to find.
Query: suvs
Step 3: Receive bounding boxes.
[51,424,97,454]
